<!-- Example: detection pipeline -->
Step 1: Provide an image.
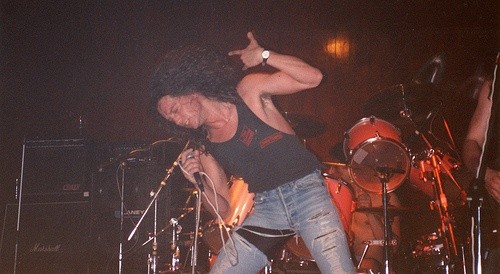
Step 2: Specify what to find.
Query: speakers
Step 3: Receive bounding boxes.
[0,138,99,274]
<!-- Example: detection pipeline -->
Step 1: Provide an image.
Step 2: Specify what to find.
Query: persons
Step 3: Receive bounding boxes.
[149,32,357,274]
[328,142,401,274]
[464,68,500,274]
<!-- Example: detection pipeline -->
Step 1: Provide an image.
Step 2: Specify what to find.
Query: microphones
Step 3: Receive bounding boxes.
[186,152,204,193]
[399,110,412,121]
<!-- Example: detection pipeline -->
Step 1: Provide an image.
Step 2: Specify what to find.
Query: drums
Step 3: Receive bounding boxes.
[202,174,255,252]
[408,145,466,202]
[285,171,356,263]
[341,115,410,195]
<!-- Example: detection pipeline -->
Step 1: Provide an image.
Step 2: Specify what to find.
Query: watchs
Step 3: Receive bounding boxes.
[261,48,270,67]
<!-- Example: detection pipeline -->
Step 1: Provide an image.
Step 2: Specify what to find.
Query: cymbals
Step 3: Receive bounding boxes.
[281,111,328,139]
